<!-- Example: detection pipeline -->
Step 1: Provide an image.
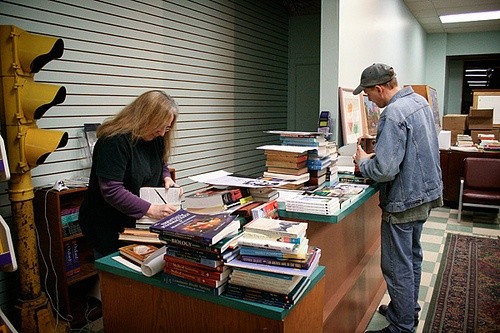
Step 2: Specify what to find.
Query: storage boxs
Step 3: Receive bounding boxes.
[441,106,500,146]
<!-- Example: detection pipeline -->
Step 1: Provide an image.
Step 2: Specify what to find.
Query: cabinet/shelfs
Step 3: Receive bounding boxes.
[33,168,175,327]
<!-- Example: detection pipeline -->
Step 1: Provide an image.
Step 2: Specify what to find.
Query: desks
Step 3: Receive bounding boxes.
[94,174,388,333]
[439,146,500,209]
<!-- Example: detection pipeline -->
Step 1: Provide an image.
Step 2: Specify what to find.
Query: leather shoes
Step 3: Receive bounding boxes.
[365,304,419,333]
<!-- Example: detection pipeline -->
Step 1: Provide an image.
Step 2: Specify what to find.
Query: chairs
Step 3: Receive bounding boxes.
[457,157,500,223]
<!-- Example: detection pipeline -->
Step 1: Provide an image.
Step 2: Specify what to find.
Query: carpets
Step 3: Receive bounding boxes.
[416,230,500,333]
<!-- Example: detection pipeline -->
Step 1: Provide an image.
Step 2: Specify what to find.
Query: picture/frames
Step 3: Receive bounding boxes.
[426,86,442,130]
[361,93,381,137]
[338,87,368,145]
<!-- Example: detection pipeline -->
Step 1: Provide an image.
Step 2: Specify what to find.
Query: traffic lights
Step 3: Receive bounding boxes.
[10,25,68,171]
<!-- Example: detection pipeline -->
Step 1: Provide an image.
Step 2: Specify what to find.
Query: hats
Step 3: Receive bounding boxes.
[352,62,397,95]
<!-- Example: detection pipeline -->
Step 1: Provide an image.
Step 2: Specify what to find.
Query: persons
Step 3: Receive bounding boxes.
[78,90,185,260]
[352,63,443,333]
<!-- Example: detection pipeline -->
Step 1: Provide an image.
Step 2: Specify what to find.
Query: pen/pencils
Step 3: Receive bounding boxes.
[154,188,167,205]
